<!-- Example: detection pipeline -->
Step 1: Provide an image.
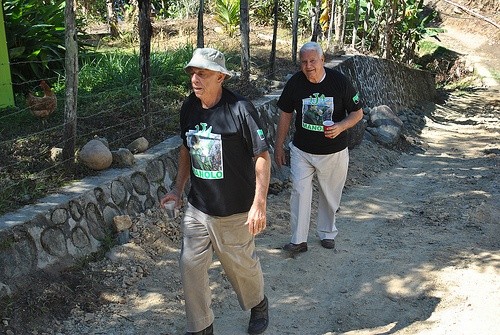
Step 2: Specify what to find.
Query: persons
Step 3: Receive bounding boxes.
[275,43,364,252]
[161,47,271,335]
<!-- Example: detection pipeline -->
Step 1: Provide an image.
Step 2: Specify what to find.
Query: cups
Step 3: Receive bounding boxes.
[164,201,176,218]
[323,121,335,136]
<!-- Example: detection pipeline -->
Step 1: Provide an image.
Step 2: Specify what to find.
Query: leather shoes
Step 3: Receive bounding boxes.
[184,324,213,335]
[283,242,307,252]
[321,239,334,249]
[248,294,269,335]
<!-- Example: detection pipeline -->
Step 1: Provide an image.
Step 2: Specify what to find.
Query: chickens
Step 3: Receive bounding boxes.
[23,80,57,125]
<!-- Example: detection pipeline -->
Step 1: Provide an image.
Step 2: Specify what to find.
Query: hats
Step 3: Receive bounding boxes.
[184,48,233,81]
